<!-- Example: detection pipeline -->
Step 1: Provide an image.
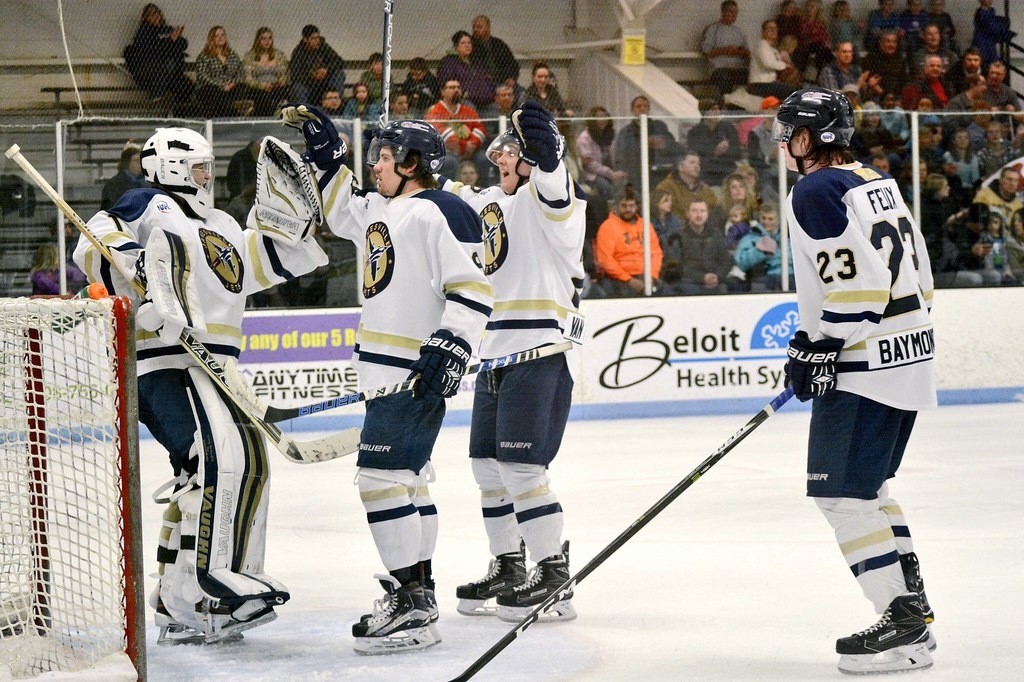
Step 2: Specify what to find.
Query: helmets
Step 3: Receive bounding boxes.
[366,119,447,175]
[770,86,856,148]
[484,126,537,167]
[140,127,216,219]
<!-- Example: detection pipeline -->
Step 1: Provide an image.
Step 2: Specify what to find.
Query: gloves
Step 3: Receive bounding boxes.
[511,100,567,173]
[281,102,348,170]
[783,331,845,402]
[406,330,476,400]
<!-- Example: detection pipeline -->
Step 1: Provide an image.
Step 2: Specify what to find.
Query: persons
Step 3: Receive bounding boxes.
[453,97,1024,289]
[278,101,495,655]
[288,23,349,83]
[769,83,942,675]
[129,1,193,103]
[197,23,251,111]
[241,25,294,92]
[369,99,590,621]
[700,1,749,84]
[225,133,260,224]
[31,239,84,295]
[99,147,148,211]
[748,1,1022,96]
[71,126,329,644]
[423,80,490,162]
[315,15,560,116]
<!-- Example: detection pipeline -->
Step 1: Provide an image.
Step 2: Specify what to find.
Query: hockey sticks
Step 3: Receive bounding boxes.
[381,1,396,135]
[5,142,360,464]
[447,378,795,682]
[224,340,575,423]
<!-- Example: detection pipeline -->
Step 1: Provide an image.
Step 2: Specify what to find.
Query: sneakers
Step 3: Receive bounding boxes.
[495,540,577,623]
[352,559,442,653]
[456,537,528,616]
[836,552,936,676]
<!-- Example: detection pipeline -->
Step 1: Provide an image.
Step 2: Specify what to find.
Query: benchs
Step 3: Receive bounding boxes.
[0,78,719,298]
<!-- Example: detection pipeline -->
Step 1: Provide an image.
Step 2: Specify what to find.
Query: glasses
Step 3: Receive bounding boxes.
[192,164,211,172]
